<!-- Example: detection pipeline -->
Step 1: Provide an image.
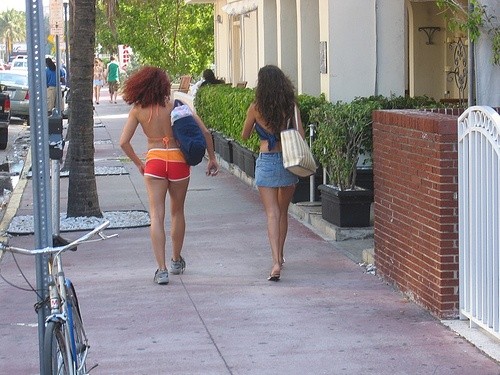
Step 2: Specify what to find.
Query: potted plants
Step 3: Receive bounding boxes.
[193,83,382,230]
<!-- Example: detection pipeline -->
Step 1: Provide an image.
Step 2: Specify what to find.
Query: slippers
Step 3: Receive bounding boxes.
[268,273,279,280]
[281,257,285,266]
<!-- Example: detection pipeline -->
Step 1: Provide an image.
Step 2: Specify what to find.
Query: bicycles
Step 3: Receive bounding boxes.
[0,221,120,375]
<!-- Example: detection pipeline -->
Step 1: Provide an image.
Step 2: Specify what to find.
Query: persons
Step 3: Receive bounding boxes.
[242,65,306,281]
[198,68,224,88]
[46,55,121,115]
[120,65,220,285]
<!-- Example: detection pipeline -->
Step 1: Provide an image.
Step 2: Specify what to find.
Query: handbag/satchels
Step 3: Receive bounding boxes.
[170,91,207,166]
[280,103,317,177]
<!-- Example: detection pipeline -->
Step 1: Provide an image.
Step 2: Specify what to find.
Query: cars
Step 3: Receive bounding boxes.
[0,54,31,123]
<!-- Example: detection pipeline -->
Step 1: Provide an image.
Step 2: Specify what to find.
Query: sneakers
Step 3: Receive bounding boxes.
[153,268,169,284]
[171,257,185,274]
